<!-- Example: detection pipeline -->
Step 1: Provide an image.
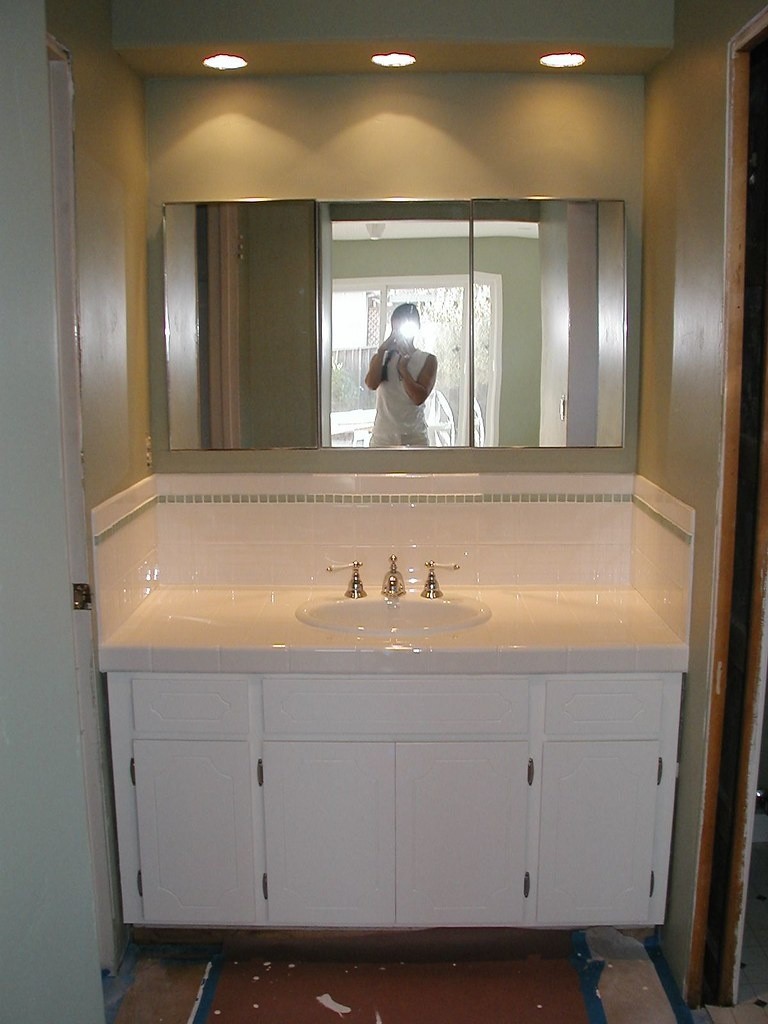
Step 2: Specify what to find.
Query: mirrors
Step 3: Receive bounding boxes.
[161,197,629,451]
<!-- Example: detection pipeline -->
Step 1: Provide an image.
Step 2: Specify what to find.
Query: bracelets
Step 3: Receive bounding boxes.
[399,375,413,382]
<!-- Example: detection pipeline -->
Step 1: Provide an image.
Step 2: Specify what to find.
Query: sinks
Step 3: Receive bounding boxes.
[295,592,493,636]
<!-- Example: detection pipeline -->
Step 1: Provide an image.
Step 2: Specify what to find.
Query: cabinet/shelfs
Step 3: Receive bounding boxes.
[98,586,688,928]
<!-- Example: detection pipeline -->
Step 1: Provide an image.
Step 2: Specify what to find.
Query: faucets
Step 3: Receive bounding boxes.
[381,554,406,597]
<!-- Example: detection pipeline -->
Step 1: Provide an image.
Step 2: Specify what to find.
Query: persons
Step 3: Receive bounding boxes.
[364,303,439,447]
